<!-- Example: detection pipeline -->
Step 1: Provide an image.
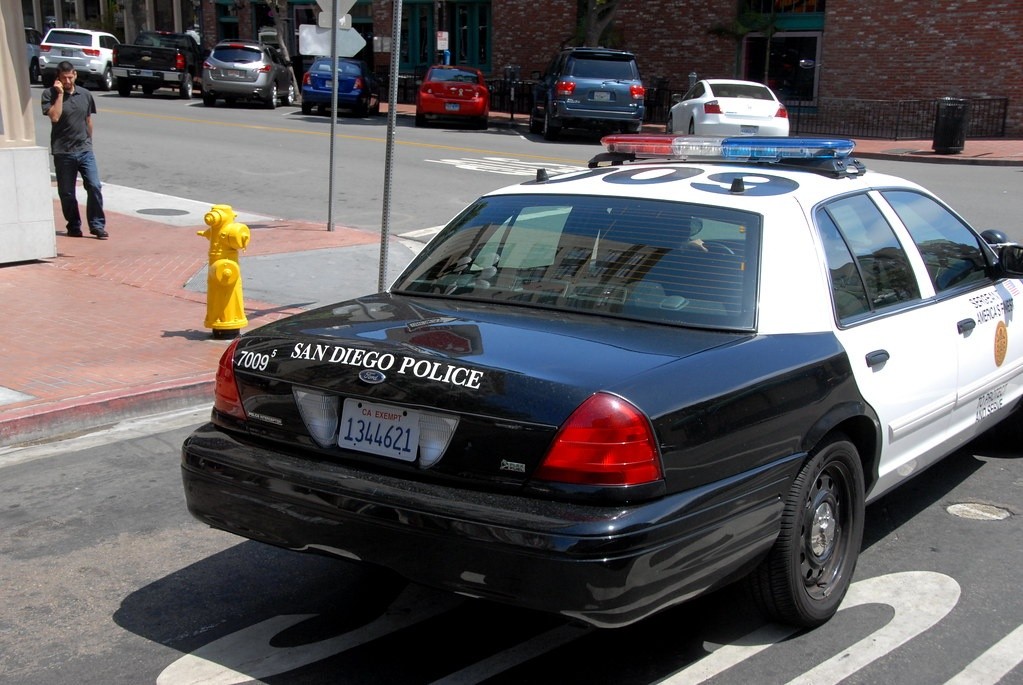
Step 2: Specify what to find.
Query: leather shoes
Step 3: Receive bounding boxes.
[67,226,82,237]
[90,227,109,237]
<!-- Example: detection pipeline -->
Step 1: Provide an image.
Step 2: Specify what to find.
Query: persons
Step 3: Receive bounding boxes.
[685,238,710,253]
[41,61,110,239]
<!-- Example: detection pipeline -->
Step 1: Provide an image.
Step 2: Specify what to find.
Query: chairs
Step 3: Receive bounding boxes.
[823,236,874,319]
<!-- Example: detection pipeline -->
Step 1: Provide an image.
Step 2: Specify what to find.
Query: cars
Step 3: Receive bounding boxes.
[25,27,44,85]
[182,134,1023,632]
[301,57,383,118]
[415,65,495,130]
[666,78,791,139]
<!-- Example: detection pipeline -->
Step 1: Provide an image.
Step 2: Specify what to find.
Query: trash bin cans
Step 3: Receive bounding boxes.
[930,97,971,155]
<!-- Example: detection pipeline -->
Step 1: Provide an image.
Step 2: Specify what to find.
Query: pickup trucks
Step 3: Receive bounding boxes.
[112,30,211,100]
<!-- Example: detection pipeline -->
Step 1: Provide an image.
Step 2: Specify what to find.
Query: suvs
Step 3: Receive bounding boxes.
[529,45,646,142]
[37,28,122,91]
[202,39,296,109]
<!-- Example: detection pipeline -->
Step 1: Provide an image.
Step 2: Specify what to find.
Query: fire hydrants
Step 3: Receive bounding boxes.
[194,205,250,339]
